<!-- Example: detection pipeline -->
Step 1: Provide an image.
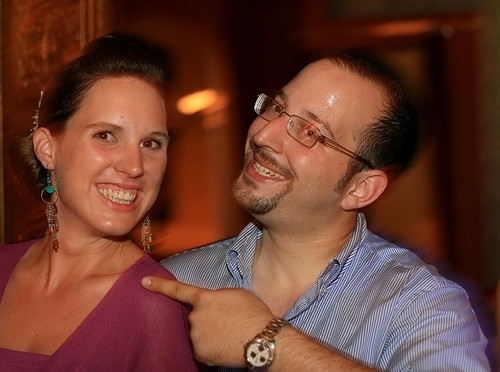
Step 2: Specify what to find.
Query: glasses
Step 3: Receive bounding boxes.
[253,93,377,171]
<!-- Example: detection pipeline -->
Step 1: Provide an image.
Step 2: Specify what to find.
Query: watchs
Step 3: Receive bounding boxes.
[243,317,291,372]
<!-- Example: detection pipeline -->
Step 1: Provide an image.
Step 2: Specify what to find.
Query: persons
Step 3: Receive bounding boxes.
[139,47,493,372]
[2,28,211,372]
